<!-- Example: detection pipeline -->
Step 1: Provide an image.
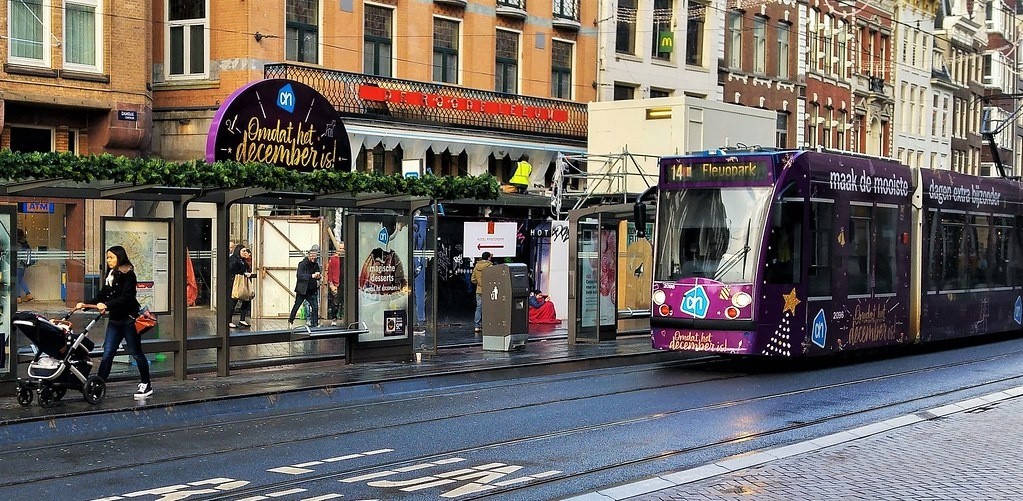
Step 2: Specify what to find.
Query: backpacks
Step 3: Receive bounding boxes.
[18,240,35,267]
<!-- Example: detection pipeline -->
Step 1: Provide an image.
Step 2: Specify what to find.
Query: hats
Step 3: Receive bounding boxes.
[482,252,490,260]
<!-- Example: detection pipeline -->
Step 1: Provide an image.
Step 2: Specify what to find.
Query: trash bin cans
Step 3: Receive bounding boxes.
[481,263,530,352]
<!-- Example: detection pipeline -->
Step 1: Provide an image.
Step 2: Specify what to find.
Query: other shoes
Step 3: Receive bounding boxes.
[331,320,337,325]
[475,327,482,331]
[238,322,251,327]
[17,297,21,303]
[305,320,311,326]
[229,325,238,328]
[24,294,33,302]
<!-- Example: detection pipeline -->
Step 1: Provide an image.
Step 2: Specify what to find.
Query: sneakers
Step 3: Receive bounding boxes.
[134,383,154,398]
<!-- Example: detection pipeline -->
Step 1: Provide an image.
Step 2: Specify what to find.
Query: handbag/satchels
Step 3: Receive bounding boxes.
[133,305,158,334]
[232,273,255,303]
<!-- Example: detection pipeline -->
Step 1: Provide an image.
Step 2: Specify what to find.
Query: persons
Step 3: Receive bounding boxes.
[246,249,251,271]
[470,252,493,332]
[76,246,154,397]
[303,245,324,326]
[528,269,534,289]
[287,249,321,328]
[932,242,1020,291]
[230,244,254,327]
[16,228,33,304]
[328,241,346,325]
[529,290,562,324]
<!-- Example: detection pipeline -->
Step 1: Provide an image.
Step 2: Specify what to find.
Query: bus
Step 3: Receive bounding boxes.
[649,141,1023,359]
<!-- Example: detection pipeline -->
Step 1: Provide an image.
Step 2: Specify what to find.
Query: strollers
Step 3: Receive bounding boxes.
[12,302,106,406]
